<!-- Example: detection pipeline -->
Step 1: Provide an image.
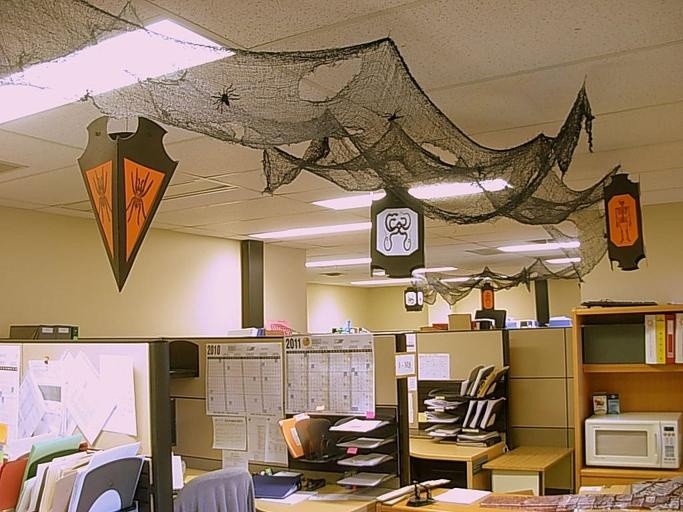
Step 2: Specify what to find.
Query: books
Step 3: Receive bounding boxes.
[422,360,510,448]
[644,314,683,365]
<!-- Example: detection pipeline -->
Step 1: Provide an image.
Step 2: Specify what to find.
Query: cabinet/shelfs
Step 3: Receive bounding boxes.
[570,305,683,495]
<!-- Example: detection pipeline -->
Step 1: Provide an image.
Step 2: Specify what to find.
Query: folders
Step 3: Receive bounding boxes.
[253,476,301,499]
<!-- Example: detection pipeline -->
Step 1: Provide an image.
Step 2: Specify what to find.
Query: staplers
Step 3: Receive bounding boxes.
[306,478,326,490]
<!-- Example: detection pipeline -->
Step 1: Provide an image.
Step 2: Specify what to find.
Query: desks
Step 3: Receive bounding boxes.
[0,326,576,512]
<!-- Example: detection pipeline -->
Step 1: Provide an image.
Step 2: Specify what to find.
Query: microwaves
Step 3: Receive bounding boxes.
[586,412,682,470]
